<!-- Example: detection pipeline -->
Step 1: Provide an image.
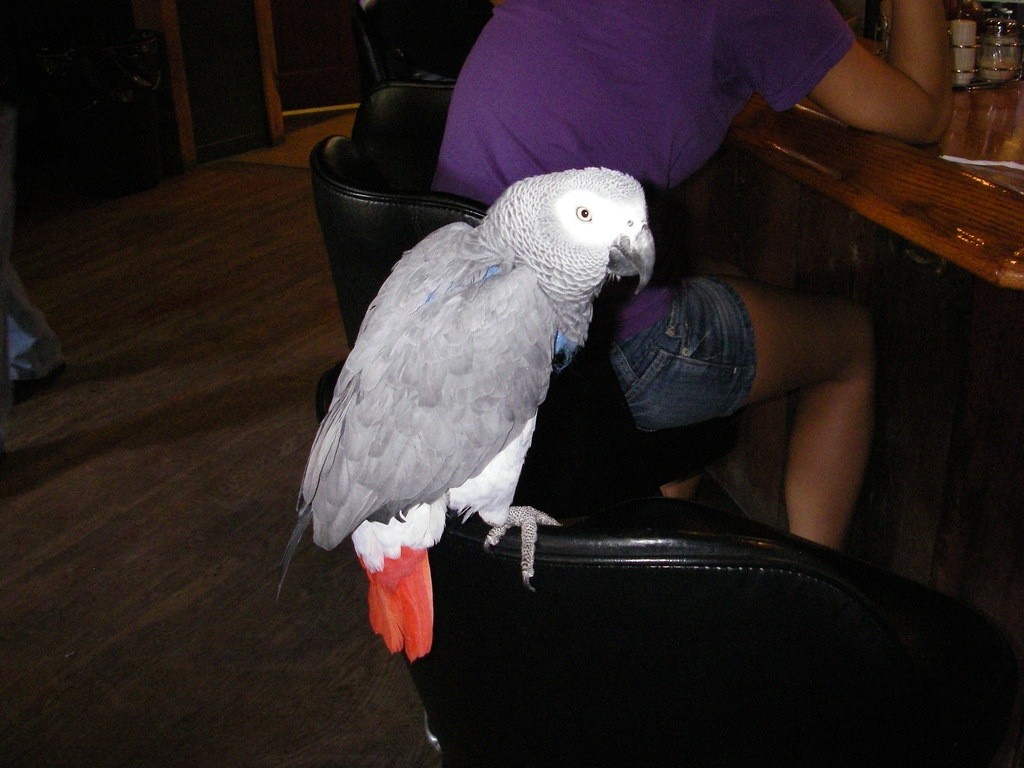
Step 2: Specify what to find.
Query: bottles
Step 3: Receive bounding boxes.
[977,16,1020,83]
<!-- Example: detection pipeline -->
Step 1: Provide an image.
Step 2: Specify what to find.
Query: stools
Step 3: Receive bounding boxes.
[310,0,1020,768]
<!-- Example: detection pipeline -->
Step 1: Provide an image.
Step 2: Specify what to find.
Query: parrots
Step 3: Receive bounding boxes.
[272,166,655,663]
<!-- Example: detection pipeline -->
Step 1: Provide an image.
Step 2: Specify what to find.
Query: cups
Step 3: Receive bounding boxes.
[874,16,890,60]
[945,15,977,87]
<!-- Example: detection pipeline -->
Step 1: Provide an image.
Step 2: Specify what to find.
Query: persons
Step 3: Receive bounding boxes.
[428,1,955,557]
[1,2,67,406]
